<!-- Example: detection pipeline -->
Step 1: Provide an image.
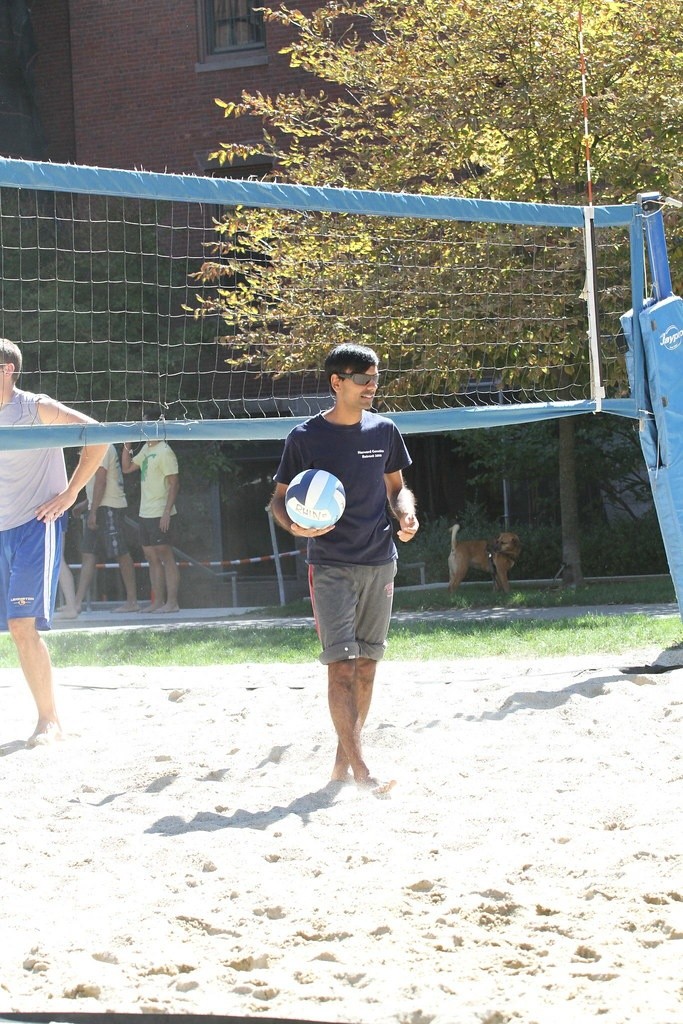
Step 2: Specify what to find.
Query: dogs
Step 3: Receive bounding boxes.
[444,524,524,591]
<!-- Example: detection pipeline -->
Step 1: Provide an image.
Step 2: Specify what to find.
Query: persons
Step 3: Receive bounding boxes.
[269,343,420,792]
[53,410,181,620]
[0,338,110,748]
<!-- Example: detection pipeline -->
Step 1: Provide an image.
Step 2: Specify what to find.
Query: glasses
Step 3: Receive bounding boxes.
[338,373,379,386]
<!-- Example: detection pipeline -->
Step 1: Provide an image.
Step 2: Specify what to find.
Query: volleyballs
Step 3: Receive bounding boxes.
[285,468,347,532]
